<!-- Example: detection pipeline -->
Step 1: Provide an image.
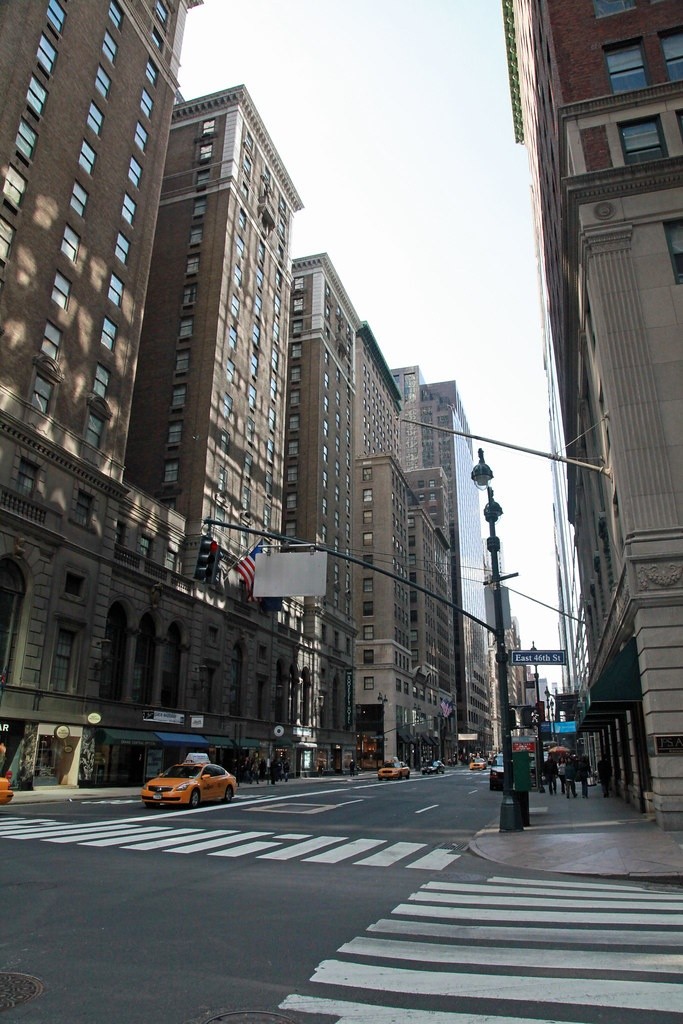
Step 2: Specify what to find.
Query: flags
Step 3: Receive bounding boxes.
[441,700,453,717]
[260,597,282,612]
[236,536,264,602]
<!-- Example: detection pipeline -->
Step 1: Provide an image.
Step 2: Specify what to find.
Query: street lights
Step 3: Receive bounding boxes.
[530,641,545,793]
[550,698,555,741]
[412,703,422,771]
[545,686,551,742]
[378,692,388,766]
[471,448,524,832]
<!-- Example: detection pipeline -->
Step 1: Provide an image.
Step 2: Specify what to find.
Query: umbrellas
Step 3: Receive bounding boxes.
[549,745,570,753]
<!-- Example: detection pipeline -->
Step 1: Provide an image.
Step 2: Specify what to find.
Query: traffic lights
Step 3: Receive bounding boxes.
[192,535,217,582]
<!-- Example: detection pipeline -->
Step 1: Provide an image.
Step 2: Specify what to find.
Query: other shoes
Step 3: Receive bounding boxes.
[554,788,557,793]
[604,792,609,798]
[573,794,577,797]
[567,795,570,798]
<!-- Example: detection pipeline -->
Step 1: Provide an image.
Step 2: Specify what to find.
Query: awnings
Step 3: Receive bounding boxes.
[204,736,233,748]
[231,739,261,750]
[95,728,163,746]
[574,637,642,732]
[154,732,209,748]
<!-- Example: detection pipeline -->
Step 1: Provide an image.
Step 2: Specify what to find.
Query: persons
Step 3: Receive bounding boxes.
[542,755,558,795]
[349,759,355,777]
[597,754,612,797]
[260,755,267,781]
[317,761,325,778]
[557,756,566,794]
[270,758,290,785]
[231,751,260,784]
[562,753,592,799]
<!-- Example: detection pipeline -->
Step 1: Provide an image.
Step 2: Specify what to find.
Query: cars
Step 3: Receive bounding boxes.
[378,757,410,781]
[421,760,446,775]
[487,753,536,791]
[470,757,486,771]
[141,753,236,808]
[0,777,14,805]
